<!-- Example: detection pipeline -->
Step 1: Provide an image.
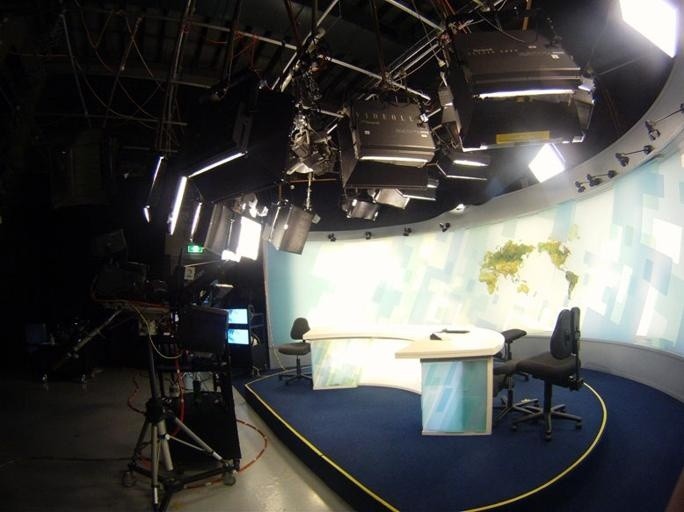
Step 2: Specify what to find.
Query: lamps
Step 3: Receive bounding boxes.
[585,103,684,186]
[144,1,595,263]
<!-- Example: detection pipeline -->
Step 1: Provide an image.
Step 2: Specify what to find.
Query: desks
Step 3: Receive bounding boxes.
[302,324,505,438]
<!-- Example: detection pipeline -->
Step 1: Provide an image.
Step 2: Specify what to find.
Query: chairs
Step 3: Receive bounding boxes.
[277,318,315,387]
[493,306,586,440]
